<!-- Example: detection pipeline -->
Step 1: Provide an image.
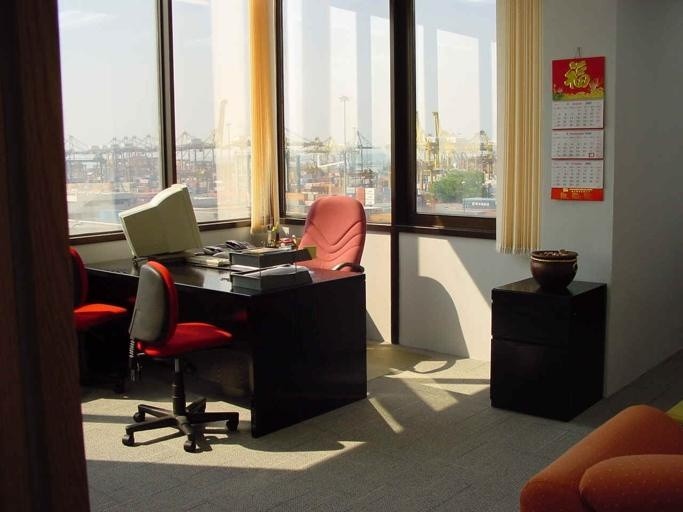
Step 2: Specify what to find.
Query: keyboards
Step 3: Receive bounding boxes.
[188,255,230,267]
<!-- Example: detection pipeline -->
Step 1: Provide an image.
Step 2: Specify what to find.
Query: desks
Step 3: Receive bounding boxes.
[81,251,369,443]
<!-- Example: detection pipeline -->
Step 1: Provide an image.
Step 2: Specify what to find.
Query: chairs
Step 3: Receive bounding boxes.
[67,246,129,390]
[522,405,681,512]
[120,258,243,452]
[287,195,370,276]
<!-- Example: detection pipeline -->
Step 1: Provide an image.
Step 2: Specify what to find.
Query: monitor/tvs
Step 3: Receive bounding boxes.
[119,182,203,261]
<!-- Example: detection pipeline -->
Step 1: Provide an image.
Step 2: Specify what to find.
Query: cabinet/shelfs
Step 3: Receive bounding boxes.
[489,272,610,421]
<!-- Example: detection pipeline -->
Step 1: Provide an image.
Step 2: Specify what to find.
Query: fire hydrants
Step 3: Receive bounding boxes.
[339,95,349,196]
